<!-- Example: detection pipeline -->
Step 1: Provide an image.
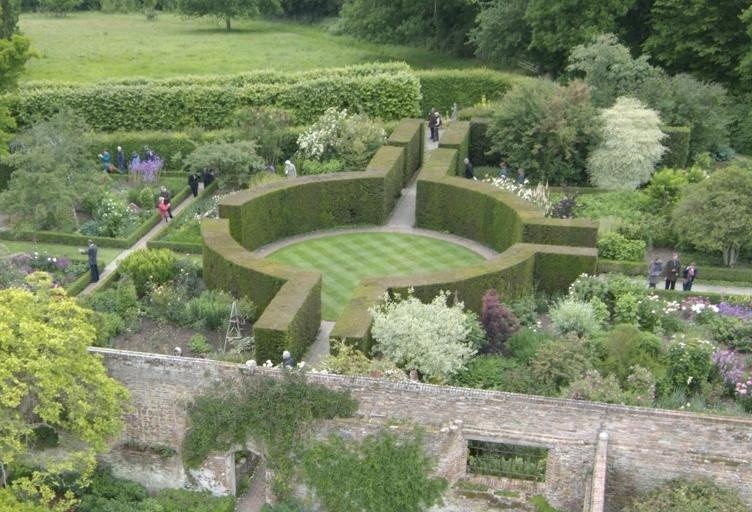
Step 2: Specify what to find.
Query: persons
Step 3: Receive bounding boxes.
[283,159,297,178]
[665,252,682,290]
[646,255,664,289]
[281,348,295,368]
[78,238,100,284]
[517,168,526,184]
[427,107,436,140]
[432,112,441,142]
[97,145,215,222]
[498,162,508,178]
[463,157,474,180]
[681,259,698,290]
